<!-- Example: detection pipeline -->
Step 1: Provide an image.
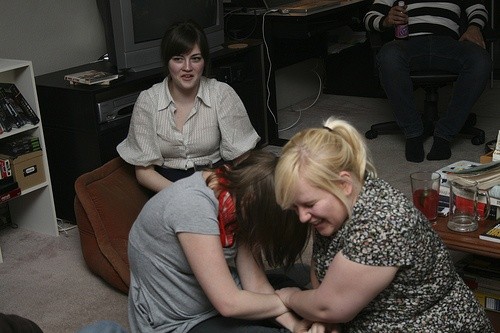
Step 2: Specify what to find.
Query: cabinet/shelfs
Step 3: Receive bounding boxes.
[0,58,59,265]
[35,34,269,226]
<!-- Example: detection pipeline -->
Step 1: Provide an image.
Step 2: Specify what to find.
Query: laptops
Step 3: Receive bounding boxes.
[263,0,340,13]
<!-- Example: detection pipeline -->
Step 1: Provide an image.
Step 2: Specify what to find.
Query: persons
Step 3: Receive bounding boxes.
[363,0,490,163]
[127,117,490,333]
[117,24,260,191]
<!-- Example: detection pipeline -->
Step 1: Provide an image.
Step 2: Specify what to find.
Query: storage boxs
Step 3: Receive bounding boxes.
[472,285,500,313]
[0,82,46,201]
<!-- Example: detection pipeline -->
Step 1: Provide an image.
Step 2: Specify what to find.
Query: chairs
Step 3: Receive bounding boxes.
[365,31,486,145]
[73,156,150,295]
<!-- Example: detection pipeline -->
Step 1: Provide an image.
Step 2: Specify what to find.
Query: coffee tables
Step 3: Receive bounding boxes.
[432,213,500,333]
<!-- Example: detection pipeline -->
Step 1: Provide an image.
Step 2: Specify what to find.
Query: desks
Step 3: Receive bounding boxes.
[223,0,375,147]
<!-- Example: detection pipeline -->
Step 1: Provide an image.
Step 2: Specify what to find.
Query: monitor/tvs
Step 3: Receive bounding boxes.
[95,0,225,72]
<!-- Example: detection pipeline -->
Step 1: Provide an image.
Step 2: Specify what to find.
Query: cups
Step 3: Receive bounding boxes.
[446,177,490,232]
[409,170,441,225]
